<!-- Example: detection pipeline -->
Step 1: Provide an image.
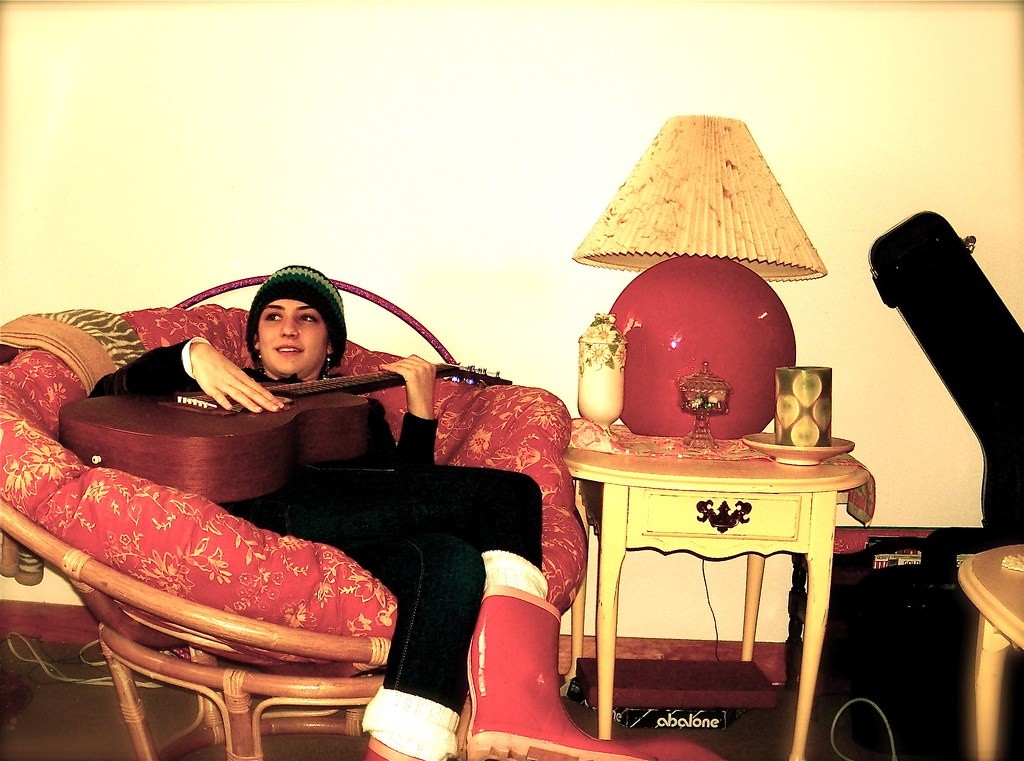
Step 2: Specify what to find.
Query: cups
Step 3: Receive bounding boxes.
[775,364,833,448]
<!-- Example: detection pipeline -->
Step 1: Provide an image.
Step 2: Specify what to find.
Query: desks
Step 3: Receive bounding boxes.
[958,543,1024,761]
[562,418,871,761]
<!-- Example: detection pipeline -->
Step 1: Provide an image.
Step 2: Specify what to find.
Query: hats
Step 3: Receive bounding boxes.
[244,266,346,372]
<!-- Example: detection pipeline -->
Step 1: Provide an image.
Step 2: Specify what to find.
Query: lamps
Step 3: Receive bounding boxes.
[573,115,831,439]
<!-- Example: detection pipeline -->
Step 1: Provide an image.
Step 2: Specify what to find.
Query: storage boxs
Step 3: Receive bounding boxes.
[577,658,778,730]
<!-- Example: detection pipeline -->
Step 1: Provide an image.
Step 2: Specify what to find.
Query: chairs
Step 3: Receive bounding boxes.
[0,307,588,761]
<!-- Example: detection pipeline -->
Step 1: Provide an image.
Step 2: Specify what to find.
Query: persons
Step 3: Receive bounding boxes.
[86,266,656,761]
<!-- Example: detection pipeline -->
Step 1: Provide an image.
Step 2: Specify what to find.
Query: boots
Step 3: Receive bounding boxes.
[362,689,460,761]
[462,585,656,761]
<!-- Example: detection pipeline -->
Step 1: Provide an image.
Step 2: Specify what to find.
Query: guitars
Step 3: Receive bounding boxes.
[56,362,514,504]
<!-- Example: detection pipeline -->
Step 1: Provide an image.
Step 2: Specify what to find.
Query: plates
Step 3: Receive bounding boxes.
[743,434,855,467]
